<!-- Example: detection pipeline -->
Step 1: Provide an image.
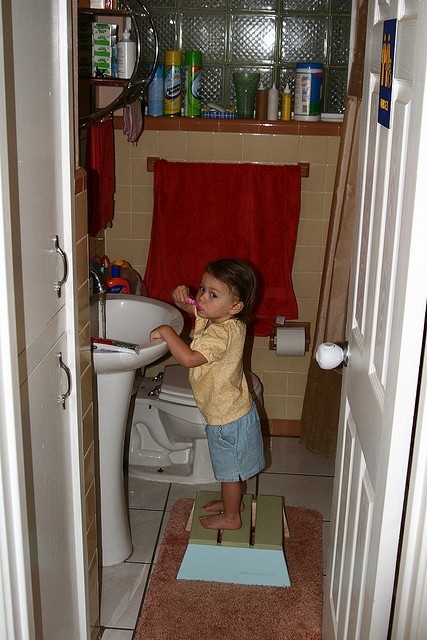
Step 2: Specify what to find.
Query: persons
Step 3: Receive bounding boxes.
[149,258,265,529]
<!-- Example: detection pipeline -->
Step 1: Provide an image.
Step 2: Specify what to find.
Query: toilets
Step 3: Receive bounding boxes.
[124,364,263,484]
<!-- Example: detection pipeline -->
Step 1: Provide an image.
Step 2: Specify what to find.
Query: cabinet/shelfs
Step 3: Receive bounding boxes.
[78,2,159,125]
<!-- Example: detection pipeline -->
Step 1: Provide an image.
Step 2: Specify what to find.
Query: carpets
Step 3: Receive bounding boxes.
[132,497,323,639]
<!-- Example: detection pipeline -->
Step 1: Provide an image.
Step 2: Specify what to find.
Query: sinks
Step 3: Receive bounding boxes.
[91,299,170,346]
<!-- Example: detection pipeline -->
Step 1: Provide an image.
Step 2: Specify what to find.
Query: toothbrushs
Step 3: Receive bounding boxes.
[185,296,203,311]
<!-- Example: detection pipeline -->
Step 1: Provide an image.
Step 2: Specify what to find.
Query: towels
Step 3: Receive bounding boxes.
[84,120,115,236]
[123,103,143,144]
[140,158,299,338]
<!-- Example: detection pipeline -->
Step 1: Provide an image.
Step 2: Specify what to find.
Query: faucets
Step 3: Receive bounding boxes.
[89,267,106,295]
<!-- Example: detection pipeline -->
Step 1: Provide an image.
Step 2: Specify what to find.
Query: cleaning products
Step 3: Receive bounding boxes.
[105,265,131,294]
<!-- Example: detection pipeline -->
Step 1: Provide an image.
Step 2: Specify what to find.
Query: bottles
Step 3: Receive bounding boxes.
[268,81,278,120]
[281,84,291,121]
[256,82,267,119]
